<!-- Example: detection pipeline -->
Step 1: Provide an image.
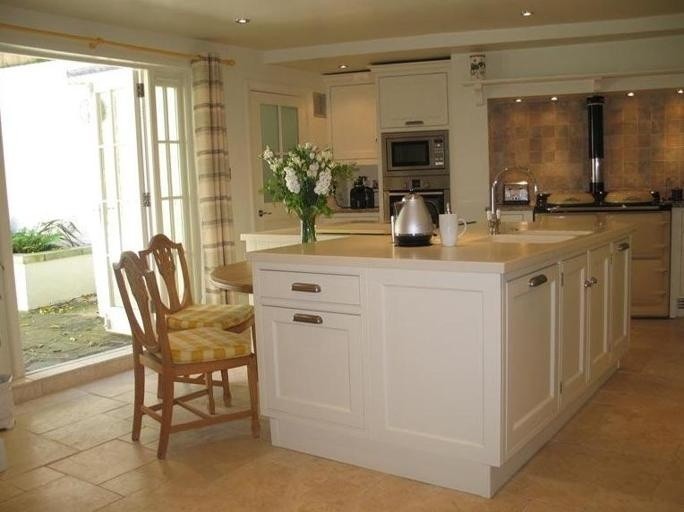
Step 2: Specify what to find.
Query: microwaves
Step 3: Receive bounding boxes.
[387,136,445,171]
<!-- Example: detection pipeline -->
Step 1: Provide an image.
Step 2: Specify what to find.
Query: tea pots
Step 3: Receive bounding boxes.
[393,189,435,246]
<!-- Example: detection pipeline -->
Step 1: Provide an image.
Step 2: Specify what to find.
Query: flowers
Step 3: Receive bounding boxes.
[258,142,356,208]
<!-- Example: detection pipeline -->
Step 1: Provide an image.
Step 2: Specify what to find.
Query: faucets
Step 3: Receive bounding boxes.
[486,166,539,235]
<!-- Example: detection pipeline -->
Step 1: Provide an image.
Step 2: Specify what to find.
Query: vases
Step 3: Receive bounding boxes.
[296,210,319,245]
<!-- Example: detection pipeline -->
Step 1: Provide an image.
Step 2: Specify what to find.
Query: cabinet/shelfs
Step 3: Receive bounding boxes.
[367,61,449,129]
[248,253,366,426]
[498,248,561,469]
[610,225,632,369]
[557,230,610,415]
[320,72,377,164]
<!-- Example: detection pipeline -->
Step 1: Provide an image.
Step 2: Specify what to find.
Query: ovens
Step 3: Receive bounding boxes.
[383,175,451,224]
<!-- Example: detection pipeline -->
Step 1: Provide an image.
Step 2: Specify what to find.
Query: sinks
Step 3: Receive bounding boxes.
[503,231,593,235]
[469,234,576,244]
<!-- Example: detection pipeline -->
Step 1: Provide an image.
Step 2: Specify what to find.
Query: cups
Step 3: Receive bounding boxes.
[438,214,467,247]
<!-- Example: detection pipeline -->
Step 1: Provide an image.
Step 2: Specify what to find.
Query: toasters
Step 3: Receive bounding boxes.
[350,188,375,209]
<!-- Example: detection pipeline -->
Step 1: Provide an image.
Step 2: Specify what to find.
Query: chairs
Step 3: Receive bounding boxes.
[112,252,260,460]
[139,234,257,406]
